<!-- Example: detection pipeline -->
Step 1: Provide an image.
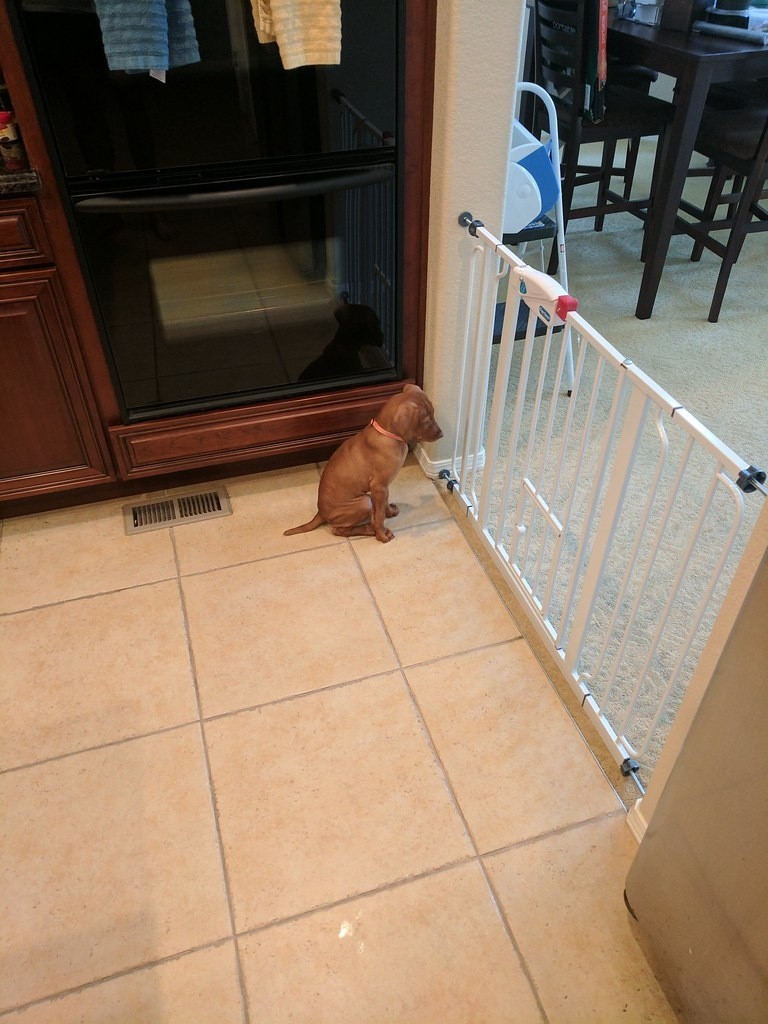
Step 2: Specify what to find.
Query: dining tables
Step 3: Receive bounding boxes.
[606,15,768,321]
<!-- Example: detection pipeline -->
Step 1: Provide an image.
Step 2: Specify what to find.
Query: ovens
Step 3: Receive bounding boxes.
[8,0,406,428]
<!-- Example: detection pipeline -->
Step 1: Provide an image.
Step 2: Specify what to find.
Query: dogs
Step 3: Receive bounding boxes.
[282,383,445,543]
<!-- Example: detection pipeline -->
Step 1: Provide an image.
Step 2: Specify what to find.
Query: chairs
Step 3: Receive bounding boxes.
[560,48,651,197]
[523,1,678,274]
[639,103,768,322]
[644,77,766,266]
